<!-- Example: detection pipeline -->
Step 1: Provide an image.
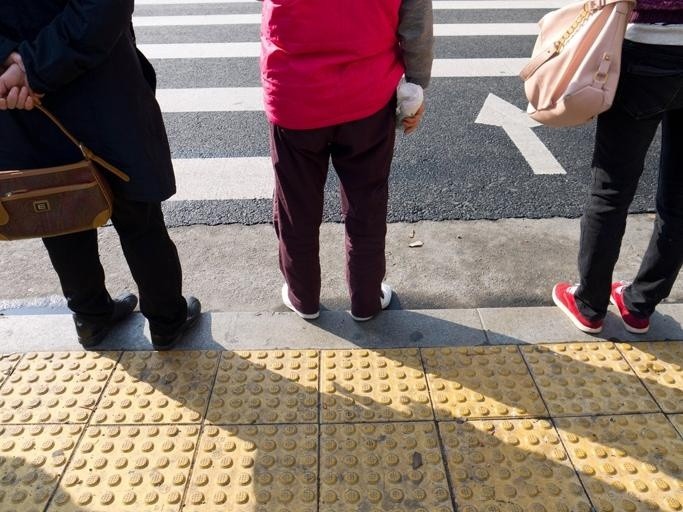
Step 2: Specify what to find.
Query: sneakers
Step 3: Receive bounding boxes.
[552,282,604,333]
[152,296,201,350]
[349,283,392,322]
[281,281,320,320]
[73,293,137,348]
[610,280,650,334]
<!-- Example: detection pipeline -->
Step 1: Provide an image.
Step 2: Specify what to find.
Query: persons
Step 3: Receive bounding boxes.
[252,0,436,323]
[0,0,203,354]
[550,1,683,338]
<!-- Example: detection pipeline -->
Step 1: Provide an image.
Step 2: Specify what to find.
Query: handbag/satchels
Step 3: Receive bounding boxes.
[0,159,113,241]
[518,0,637,128]
